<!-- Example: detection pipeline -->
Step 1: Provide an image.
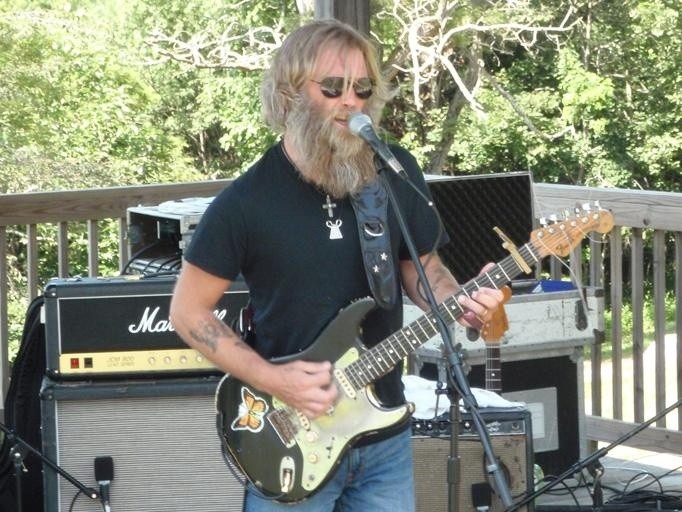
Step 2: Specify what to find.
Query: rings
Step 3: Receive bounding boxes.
[479,310,489,319]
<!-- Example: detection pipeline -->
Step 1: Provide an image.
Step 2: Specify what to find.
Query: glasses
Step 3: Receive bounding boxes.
[310,76,372,99]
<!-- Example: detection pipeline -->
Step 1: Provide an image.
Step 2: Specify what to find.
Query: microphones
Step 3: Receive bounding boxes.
[93,455,116,512]
[470,481,492,512]
[346,112,409,183]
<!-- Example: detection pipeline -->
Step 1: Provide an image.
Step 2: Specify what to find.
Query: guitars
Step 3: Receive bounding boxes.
[214,198,614,505]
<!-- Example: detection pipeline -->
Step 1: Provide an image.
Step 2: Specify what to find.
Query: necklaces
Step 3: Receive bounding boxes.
[279,142,347,219]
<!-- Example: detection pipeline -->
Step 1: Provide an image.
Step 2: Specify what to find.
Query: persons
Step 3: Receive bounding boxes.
[170,17,505,511]
